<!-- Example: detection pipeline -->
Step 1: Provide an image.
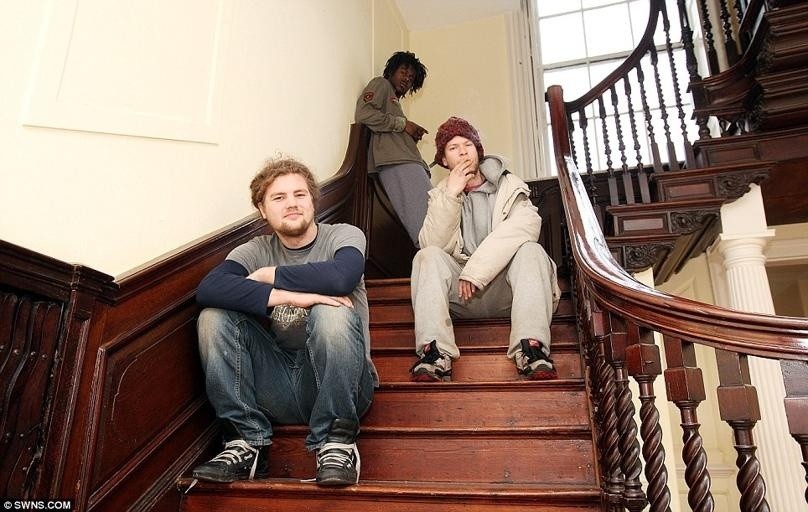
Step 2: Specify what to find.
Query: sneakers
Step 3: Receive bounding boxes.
[516,339,557,379]
[412,340,451,381]
[192,438,269,483]
[316,418,357,485]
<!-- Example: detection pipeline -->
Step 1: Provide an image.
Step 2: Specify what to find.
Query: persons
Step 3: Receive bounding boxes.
[352,48,434,251]
[410,113,564,381]
[190,155,381,487]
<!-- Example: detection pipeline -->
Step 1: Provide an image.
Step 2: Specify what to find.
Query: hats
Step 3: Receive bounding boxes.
[434,115,485,166]
